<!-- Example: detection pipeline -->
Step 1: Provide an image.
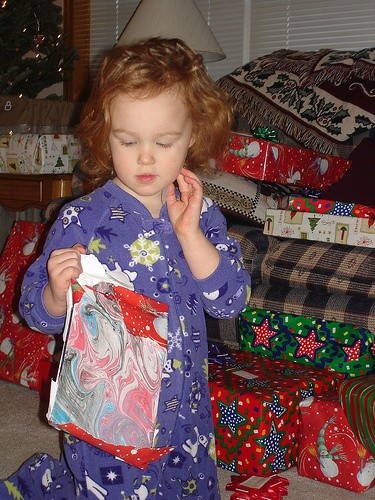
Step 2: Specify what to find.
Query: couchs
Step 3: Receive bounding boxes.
[203,48,375,370]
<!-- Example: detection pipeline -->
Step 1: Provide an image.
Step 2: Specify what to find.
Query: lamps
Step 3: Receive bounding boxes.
[112,1,225,66]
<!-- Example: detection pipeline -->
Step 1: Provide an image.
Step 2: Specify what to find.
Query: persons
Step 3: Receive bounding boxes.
[1,38,252,500]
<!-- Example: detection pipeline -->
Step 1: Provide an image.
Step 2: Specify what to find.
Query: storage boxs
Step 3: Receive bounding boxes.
[295,375,375,493]
[260,208,375,250]
[0,133,82,176]
[204,352,349,480]
[1,220,68,395]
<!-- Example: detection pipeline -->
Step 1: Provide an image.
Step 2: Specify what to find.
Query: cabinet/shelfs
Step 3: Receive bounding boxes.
[1,175,92,219]
